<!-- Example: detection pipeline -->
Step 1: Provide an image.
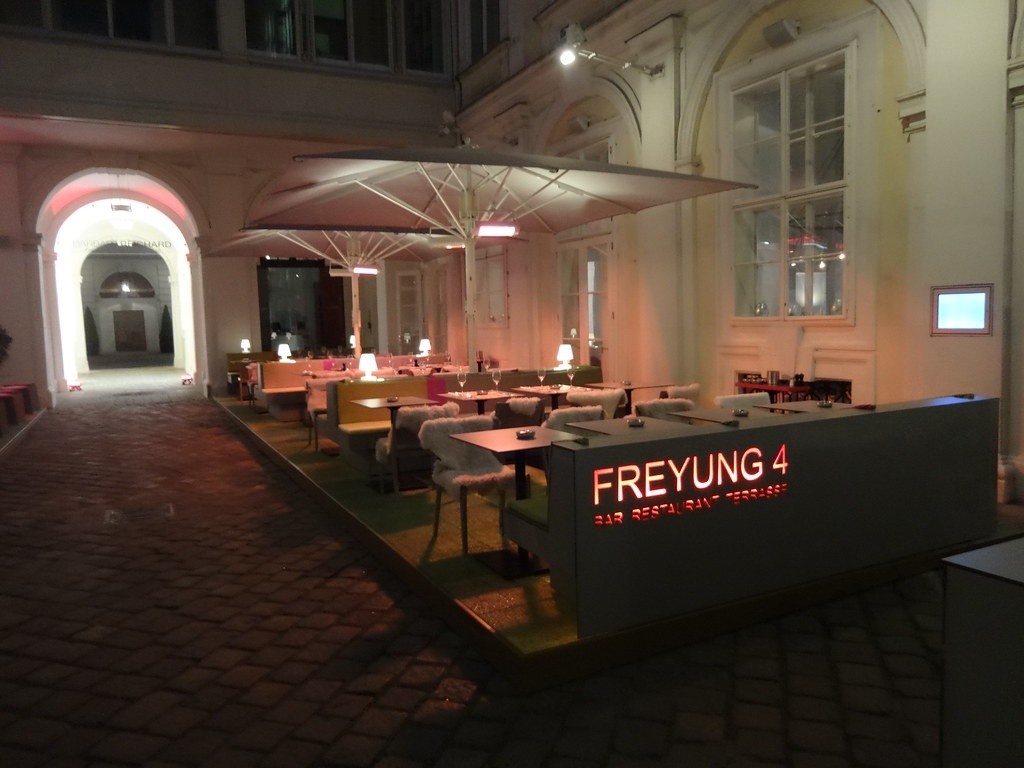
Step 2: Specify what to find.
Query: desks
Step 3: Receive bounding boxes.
[463,359,499,373]
[586,381,675,415]
[565,415,694,435]
[448,425,578,582]
[666,405,785,424]
[735,381,812,412]
[230,359,261,401]
[510,384,593,411]
[752,400,855,412]
[437,389,526,416]
[349,395,440,495]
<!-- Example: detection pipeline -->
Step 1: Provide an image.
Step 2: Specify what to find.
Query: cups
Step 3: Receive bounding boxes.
[409,352,414,361]
[371,348,376,355]
[443,362,453,372]
[420,361,427,374]
[308,350,314,359]
[322,345,328,354]
[338,345,343,354]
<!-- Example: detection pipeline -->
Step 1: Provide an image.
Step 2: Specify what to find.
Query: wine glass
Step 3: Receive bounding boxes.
[457,372,467,395]
[566,364,576,389]
[492,369,502,394]
[537,367,546,390]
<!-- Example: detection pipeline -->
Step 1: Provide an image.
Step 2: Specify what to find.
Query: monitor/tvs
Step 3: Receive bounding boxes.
[932,287,990,334]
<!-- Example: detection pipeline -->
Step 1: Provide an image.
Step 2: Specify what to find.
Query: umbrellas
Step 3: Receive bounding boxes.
[193,137,759,371]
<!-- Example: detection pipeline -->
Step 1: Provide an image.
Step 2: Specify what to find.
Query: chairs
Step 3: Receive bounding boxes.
[658,383,701,399]
[633,397,695,424]
[539,404,610,483]
[305,377,346,446]
[418,413,532,566]
[490,395,546,465]
[567,389,628,421]
[374,401,460,506]
[713,393,771,413]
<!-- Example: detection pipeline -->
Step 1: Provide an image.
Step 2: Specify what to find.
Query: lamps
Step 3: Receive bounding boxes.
[559,40,665,81]
[359,353,378,382]
[352,264,382,275]
[428,237,466,250]
[277,344,292,363]
[557,344,574,365]
[330,268,353,277]
[472,220,520,237]
[419,339,432,355]
[240,338,251,354]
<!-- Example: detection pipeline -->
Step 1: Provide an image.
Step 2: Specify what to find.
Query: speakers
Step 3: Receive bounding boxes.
[568,114,589,133]
[764,18,799,48]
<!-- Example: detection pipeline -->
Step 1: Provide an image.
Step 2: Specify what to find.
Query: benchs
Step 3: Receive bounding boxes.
[335,366,603,476]
[261,353,452,422]
[252,363,262,400]
[315,368,555,442]
